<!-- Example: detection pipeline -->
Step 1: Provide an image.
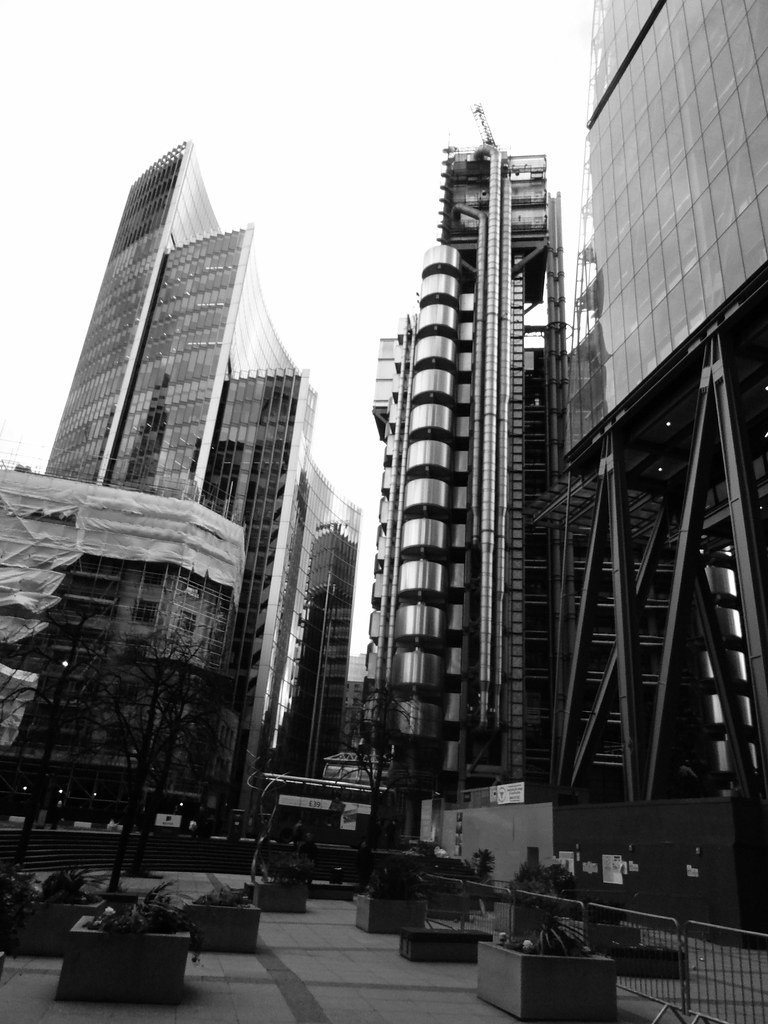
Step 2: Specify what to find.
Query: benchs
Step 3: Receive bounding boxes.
[608,941,685,980]
[399,927,493,962]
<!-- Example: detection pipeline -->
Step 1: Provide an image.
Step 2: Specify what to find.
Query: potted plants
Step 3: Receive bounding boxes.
[254,831,319,914]
[476,902,619,1022]
[356,854,438,934]
[0,859,45,978]
[53,880,204,1005]
[18,864,109,957]
[558,900,640,954]
[492,862,575,934]
[183,886,262,953]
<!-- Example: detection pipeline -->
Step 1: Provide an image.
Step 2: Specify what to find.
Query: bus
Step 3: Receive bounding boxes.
[247,773,399,854]
